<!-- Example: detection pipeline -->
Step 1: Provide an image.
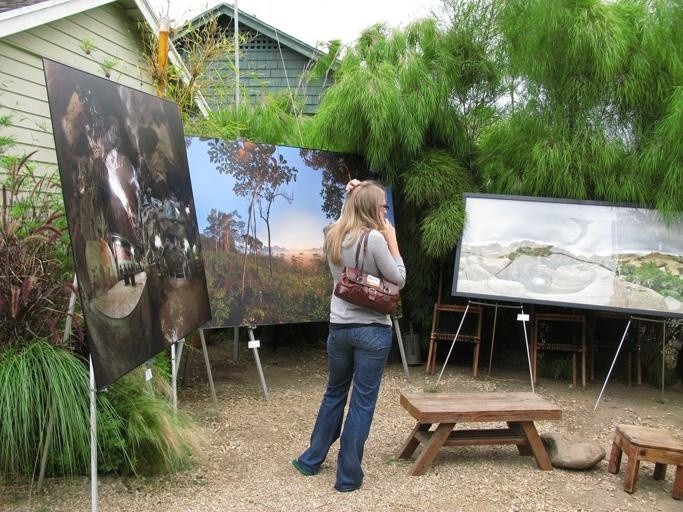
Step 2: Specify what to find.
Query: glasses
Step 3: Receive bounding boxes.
[380,204,389,210]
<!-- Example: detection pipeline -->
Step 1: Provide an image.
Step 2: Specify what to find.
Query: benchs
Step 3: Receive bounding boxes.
[394,390,563,474]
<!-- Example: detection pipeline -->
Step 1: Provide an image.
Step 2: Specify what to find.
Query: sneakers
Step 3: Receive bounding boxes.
[292,460,316,476]
[337,470,364,492]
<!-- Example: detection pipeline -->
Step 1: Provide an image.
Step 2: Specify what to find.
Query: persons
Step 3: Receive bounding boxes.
[291,178,407,492]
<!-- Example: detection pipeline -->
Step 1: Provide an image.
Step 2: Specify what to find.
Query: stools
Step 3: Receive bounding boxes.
[606,425,682,499]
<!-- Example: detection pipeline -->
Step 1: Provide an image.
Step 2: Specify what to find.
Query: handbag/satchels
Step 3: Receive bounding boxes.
[332,228,399,315]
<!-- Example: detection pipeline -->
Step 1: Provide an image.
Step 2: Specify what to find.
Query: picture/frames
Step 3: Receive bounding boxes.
[450,191,682,318]
